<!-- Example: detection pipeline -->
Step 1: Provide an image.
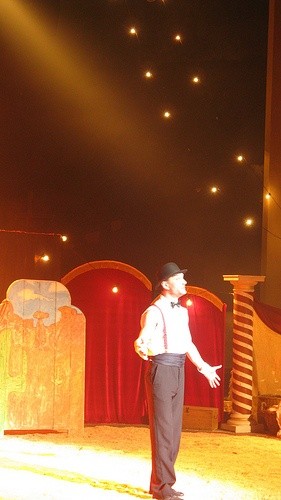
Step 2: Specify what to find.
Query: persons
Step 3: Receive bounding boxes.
[134,263,223,500]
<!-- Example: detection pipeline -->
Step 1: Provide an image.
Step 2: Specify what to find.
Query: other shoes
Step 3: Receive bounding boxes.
[152,488,183,500]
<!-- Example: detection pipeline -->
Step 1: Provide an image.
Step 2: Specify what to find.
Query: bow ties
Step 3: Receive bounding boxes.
[171,302,180,308]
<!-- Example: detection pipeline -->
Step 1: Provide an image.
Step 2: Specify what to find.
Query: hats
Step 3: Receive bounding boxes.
[154,262,188,290]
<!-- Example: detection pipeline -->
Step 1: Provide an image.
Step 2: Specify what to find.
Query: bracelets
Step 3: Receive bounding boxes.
[197,362,211,375]
